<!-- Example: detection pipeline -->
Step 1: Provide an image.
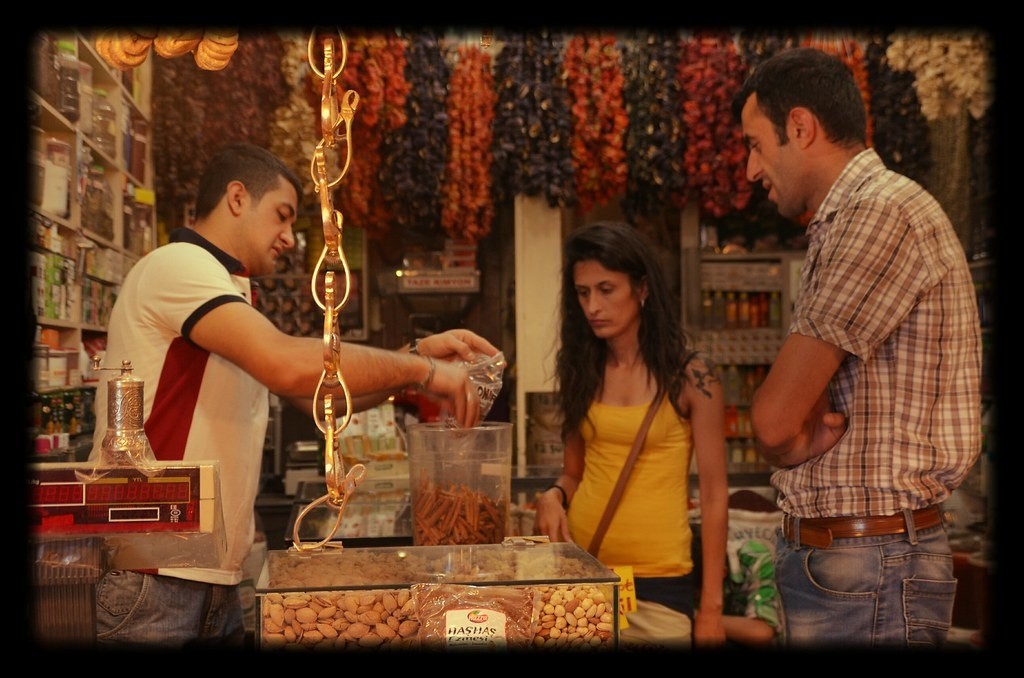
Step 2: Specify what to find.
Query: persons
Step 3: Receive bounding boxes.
[683,521,785,642]
[85,145,508,644]
[742,47,981,642]
[534,220,727,644]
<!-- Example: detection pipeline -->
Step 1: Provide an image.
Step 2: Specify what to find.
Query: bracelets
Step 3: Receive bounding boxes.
[414,355,435,389]
[409,338,421,355]
[545,485,567,509]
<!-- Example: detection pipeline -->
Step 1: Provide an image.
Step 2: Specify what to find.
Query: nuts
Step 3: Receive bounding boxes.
[260,552,616,646]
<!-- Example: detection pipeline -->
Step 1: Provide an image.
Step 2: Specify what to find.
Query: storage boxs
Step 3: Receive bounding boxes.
[252,541,622,652]
[725,486,786,574]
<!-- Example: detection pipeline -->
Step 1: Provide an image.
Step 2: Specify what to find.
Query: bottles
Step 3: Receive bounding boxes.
[33,35,156,394]
[695,289,784,480]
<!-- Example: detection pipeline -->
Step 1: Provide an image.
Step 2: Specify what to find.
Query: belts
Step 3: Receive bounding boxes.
[780,504,941,549]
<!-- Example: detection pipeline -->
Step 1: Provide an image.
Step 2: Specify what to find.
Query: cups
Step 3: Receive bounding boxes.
[406,420,514,544]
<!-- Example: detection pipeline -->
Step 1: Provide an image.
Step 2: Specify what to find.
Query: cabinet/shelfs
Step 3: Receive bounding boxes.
[30,41,159,398]
